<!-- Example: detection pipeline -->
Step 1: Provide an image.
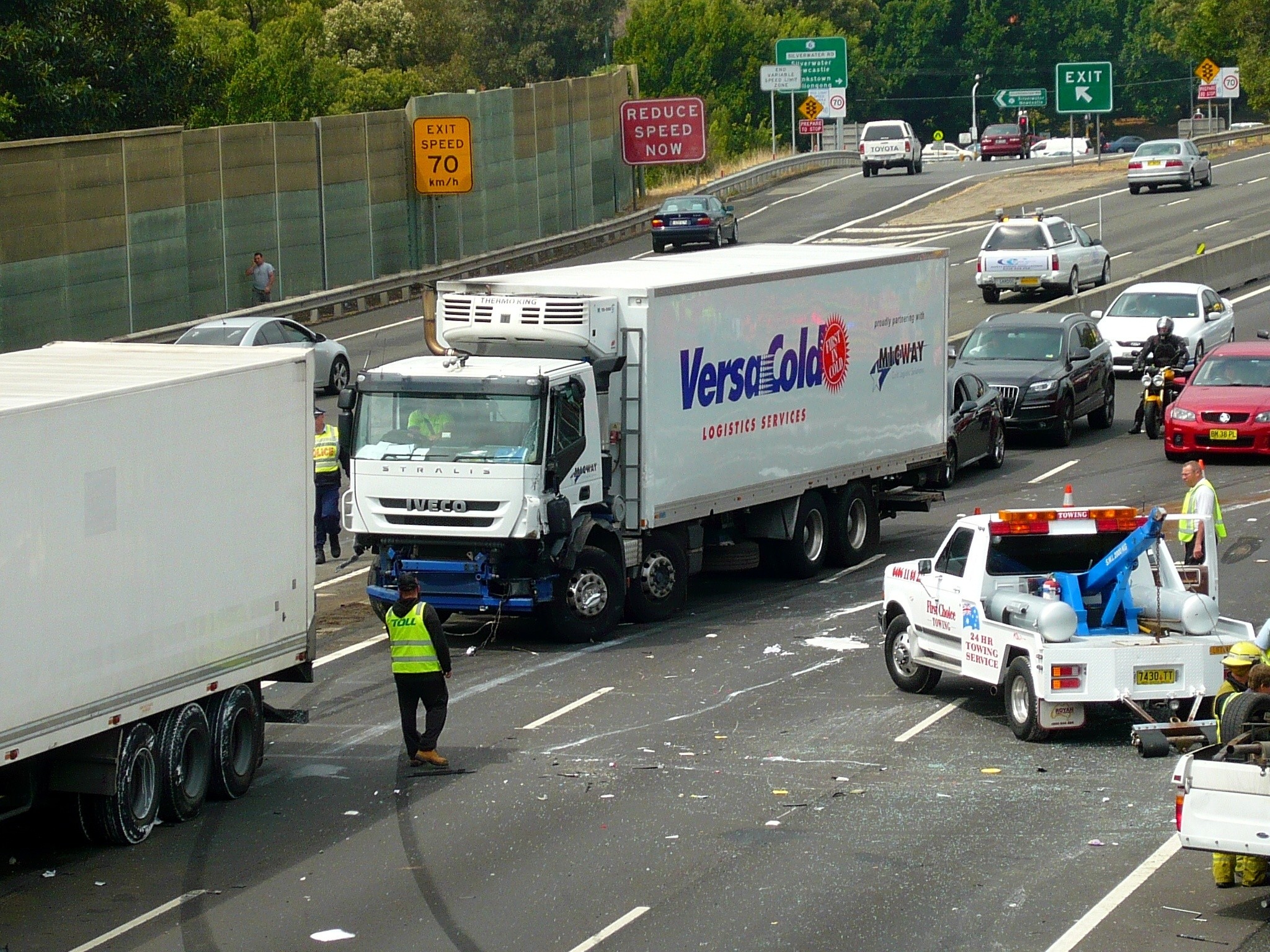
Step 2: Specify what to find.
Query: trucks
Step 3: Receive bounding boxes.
[1,337,320,851]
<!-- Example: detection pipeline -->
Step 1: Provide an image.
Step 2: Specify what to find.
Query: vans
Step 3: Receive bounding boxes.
[1031,137,1094,158]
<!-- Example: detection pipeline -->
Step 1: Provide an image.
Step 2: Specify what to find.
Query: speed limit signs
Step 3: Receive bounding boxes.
[412,117,476,196]
[1224,75,1239,89]
[831,95,845,110]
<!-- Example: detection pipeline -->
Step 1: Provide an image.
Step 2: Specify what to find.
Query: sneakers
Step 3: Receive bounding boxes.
[415,749,449,766]
[410,759,422,766]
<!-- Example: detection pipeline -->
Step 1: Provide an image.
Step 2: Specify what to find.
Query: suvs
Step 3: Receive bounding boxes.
[946,311,1117,446]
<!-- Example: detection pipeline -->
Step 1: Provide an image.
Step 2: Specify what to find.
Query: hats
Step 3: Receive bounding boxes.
[314,406,325,415]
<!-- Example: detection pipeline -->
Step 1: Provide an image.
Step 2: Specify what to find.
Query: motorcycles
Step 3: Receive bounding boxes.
[1128,350,1188,440]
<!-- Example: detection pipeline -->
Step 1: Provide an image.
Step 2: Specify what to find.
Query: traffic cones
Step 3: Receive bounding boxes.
[1060,485,1076,508]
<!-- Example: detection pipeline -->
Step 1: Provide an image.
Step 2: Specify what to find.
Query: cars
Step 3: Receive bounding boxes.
[979,123,1032,161]
[1104,135,1147,153]
[175,315,351,397]
[1229,122,1265,144]
[965,143,981,152]
[1091,281,1237,376]
[1163,341,1270,460]
[975,207,1111,304]
[859,120,923,177]
[1127,137,1212,195]
[651,195,739,254]
[922,143,974,161]
[881,368,1008,490]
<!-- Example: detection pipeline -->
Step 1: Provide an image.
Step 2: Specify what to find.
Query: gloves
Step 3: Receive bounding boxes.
[1132,361,1140,370]
[1177,363,1184,368]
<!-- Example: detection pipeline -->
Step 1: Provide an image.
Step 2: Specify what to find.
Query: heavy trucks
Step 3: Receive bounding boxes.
[340,242,951,645]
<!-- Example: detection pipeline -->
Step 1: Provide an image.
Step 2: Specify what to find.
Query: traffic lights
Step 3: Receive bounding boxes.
[1019,116,1029,134]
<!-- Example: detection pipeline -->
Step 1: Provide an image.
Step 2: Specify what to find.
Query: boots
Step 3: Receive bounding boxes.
[1129,422,1141,433]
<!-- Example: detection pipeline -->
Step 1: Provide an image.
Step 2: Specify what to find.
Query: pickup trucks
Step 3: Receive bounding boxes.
[1170,740,1270,857]
[876,504,1259,745]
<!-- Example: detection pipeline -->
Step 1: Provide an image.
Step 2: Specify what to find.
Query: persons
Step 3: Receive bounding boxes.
[1127,316,1188,434]
[1211,617,1270,888]
[1128,296,1160,316]
[311,408,350,565]
[246,252,275,306]
[384,574,453,768]
[407,399,454,442]
[1178,461,1227,565]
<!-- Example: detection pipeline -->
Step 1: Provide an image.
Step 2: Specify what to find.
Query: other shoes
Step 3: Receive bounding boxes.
[329,534,341,557]
[315,548,326,564]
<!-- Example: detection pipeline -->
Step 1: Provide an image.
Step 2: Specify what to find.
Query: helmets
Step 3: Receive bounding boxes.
[1157,316,1174,339]
[1220,643,1261,665]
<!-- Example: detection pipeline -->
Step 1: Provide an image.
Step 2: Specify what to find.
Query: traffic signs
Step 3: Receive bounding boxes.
[775,36,849,93]
[994,88,1048,107]
